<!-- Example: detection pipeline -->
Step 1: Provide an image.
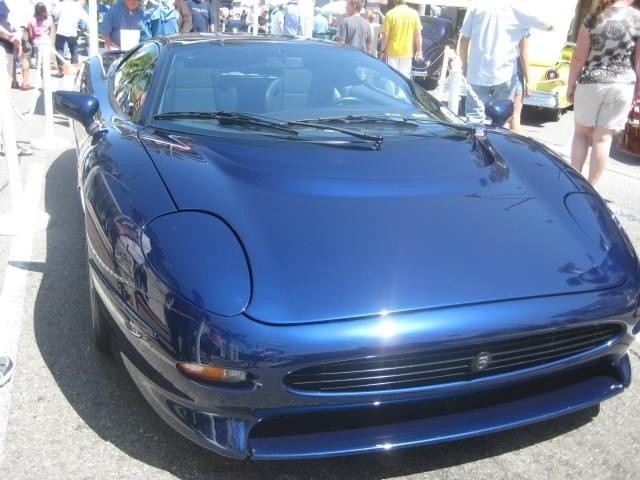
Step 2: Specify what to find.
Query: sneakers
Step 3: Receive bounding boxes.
[11,71,67,90]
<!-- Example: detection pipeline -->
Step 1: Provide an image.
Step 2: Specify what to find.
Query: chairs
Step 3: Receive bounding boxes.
[158,56,342,118]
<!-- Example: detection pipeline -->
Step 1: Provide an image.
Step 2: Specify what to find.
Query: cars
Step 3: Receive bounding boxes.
[520,43,576,121]
[411,14,457,88]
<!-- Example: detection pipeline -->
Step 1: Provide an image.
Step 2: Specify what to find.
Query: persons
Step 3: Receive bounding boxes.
[509,30,530,132]
[0,0,22,84]
[456,0,556,135]
[28,1,56,94]
[564,0,640,189]
[3,0,35,90]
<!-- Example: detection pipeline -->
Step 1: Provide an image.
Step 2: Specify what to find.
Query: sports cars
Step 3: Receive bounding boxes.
[50,31,640,462]
